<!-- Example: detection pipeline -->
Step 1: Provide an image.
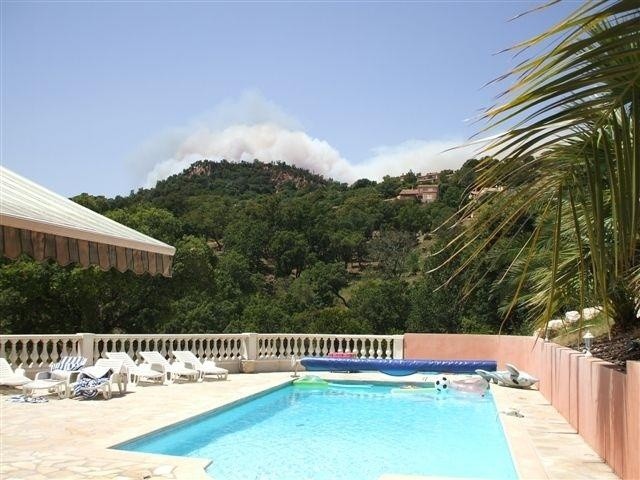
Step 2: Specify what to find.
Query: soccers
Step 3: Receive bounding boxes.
[434,375,451,392]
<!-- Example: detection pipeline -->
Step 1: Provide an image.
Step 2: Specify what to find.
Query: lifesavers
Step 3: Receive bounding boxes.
[292,376,328,388]
[450,376,488,393]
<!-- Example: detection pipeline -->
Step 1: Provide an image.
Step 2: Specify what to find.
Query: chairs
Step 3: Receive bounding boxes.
[0,354,32,394]
[104,350,167,391]
[22,354,88,405]
[63,356,128,403]
[171,348,231,383]
[138,350,201,387]
